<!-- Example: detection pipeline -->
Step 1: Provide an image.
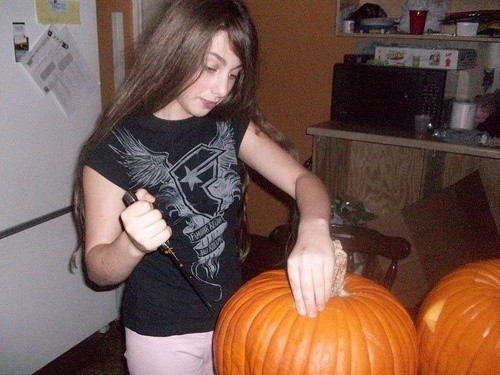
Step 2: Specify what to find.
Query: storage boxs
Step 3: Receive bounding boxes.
[375,46,478,68]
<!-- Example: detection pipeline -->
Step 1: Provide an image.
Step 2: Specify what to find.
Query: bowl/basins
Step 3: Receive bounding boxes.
[451,102,477,129]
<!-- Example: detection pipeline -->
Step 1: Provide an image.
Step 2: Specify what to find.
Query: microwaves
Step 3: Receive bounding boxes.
[330,62,449,132]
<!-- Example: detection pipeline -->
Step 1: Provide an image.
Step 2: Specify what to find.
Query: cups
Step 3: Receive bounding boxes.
[344,21,354,33]
[408,9,428,34]
[414,115,431,139]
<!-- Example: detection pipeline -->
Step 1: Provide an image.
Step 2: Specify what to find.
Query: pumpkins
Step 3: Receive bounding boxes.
[416,257,500,375]
[212,246,420,375]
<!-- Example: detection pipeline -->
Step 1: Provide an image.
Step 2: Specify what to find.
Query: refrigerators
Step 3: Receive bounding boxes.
[1,0,124,375]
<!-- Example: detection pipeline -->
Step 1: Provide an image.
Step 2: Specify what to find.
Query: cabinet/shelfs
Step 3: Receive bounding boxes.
[306,121,500,323]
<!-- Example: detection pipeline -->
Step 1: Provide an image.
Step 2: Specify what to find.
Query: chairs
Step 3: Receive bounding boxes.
[255,222,411,292]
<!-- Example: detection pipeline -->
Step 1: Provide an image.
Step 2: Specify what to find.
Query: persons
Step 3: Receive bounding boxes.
[69,0,337,375]
